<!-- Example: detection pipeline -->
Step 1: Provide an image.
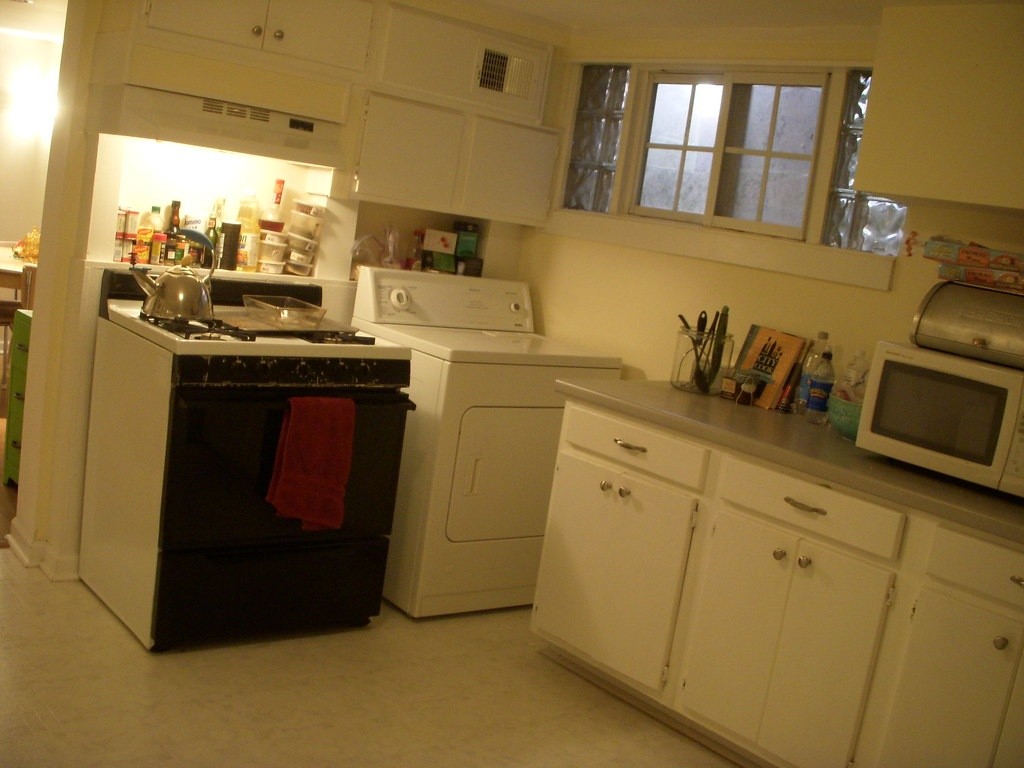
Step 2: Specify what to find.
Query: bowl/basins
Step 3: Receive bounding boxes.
[242,294,326,329]
[826,393,861,442]
[257,199,325,277]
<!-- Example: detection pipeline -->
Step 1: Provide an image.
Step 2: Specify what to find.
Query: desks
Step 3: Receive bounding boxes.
[0,247,35,302]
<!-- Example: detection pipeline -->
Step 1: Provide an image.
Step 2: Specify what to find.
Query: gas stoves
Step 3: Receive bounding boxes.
[97,267,412,389]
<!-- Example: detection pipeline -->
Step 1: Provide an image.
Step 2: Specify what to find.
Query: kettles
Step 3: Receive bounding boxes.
[129,229,219,323]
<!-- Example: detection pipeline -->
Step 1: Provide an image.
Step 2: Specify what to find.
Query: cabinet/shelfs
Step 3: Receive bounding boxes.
[129,0,375,76]
[674,453,906,768]
[330,89,564,229]
[875,518,1024,768]
[3,309,33,486]
[529,399,709,707]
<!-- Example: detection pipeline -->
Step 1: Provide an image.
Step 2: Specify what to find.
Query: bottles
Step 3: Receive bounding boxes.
[133,199,260,271]
[257,178,286,240]
[405,230,424,272]
[804,350,835,425]
[796,332,829,415]
[669,326,733,396]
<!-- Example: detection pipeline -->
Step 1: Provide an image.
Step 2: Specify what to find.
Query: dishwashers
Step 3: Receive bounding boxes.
[350,266,622,618]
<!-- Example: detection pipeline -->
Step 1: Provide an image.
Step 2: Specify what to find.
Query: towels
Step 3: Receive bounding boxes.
[266,396,355,532]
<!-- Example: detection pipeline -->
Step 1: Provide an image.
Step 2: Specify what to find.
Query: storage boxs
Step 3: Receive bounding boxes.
[421,221,484,276]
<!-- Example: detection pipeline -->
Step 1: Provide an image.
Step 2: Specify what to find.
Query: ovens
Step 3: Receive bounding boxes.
[76,382,418,654]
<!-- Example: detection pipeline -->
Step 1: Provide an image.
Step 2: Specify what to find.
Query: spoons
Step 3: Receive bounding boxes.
[677,314,710,393]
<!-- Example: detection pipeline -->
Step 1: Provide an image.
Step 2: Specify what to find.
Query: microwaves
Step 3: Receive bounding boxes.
[854,340,1024,498]
